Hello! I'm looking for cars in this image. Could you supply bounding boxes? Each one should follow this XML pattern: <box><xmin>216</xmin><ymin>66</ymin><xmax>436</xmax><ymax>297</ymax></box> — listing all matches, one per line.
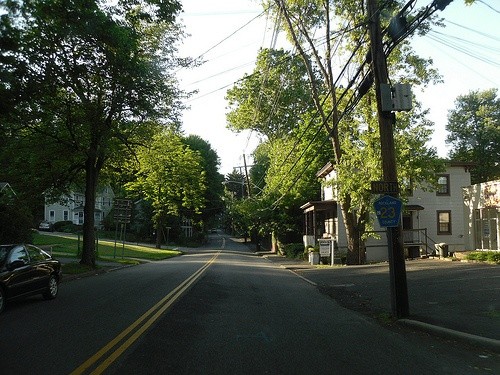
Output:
<box><xmin>0</xmin><ymin>244</ymin><xmax>61</xmax><ymax>310</ymax></box>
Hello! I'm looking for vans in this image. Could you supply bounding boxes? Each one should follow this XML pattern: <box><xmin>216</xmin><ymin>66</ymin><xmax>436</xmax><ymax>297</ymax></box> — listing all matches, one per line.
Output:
<box><xmin>212</xmin><ymin>228</ymin><xmax>217</xmax><ymax>233</ymax></box>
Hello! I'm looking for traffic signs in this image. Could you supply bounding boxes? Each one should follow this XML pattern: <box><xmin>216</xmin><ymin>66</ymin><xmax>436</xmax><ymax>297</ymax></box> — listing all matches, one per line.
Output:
<box><xmin>373</xmin><ymin>195</ymin><xmax>403</xmax><ymax>228</ymax></box>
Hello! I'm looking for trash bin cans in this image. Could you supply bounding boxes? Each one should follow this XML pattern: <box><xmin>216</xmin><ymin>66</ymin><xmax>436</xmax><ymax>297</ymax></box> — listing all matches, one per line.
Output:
<box><xmin>311</xmin><ymin>251</ymin><xmax>319</xmax><ymax>265</ymax></box>
<box><xmin>435</xmin><ymin>242</ymin><xmax>448</xmax><ymax>258</ymax></box>
